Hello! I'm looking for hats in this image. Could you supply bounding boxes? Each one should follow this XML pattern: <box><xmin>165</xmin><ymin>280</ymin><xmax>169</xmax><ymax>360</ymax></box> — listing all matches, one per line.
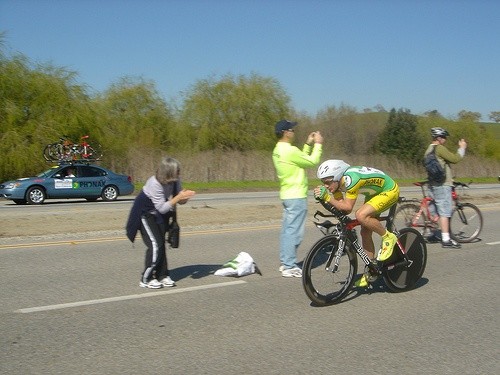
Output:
<box><xmin>275</xmin><ymin>119</ymin><xmax>298</xmax><ymax>134</ymax></box>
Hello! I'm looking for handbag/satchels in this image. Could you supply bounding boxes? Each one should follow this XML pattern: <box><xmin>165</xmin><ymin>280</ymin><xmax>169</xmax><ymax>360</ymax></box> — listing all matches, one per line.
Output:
<box><xmin>168</xmin><ymin>221</ymin><xmax>179</xmax><ymax>248</ymax></box>
<box><xmin>214</xmin><ymin>252</ymin><xmax>262</xmax><ymax>277</ymax></box>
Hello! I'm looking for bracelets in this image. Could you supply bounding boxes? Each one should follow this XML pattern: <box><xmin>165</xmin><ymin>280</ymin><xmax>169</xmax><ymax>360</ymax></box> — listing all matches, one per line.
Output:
<box><xmin>305</xmin><ymin>143</ymin><xmax>311</xmax><ymax>146</ymax></box>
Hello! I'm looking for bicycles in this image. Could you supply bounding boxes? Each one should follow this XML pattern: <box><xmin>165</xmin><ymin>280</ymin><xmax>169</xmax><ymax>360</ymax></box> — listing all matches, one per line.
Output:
<box><xmin>300</xmin><ymin>191</ymin><xmax>427</xmax><ymax>308</ymax></box>
<box><xmin>43</xmin><ymin>133</ymin><xmax>102</xmax><ymax>163</ymax></box>
<box><xmin>392</xmin><ymin>181</ymin><xmax>483</xmax><ymax>244</ymax></box>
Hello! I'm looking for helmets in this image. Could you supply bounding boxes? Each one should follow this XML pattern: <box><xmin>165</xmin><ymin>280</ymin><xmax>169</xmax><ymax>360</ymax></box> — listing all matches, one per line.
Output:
<box><xmin>317</xmin><ymin>160</ymin><xmax>351</xmax><ymax>182</ymax></box>
<box><xmin>431</xmin><ymin>127</ymin><xmax>449</xmax><ymax>138</ymax></box>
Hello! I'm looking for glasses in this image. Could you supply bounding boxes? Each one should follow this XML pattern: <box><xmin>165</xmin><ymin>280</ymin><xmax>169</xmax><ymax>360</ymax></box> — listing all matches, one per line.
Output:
<box><xmin>439</xmin><ymin>136</ymin><xmax>446</xmax><ymax>139</ymax></box>
<box><xmin>288</xmin><ymin>128</ymin><xmax>296</xmax><ymax>132</ymax></box>
<box><xmin>322</xmin><ymin>180</ymin><xmax>333</xmax><ymax>185</ymax></box>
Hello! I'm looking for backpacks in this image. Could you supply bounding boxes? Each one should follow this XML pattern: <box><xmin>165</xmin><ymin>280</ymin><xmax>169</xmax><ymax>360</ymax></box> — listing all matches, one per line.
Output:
<box><xmin>424</xmin><ymin>145</ymin><xmax>446</xmax><ymax>187</ymax></box>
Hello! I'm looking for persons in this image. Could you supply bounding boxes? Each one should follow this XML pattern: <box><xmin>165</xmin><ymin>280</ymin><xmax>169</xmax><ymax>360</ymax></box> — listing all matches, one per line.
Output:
<box><xmin>67</xmin><ymin>169</ymin><xmax>75</xmax><ymax>178</ymax></box>
<box><xmin>423</xmin><ymin>127</ymin><xmax>467</xmax><ymax>249</ymax></box>
<box><xmin>126</xmin><ymin>158</ymin><xmax>197</xmax><ymax>289</ymax></box>
<box><xmin>271</xmin><ymin>120</ymin><xmax>322</xmax><ymax>278</ymax></box>
<box><xmin>314</xmin><ymin>159</ymin><xmax>399</xmax><ymax>288</ymax></box>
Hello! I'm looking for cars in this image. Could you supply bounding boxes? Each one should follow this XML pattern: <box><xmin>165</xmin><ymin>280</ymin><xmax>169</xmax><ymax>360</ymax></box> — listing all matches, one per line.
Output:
<box><xmin>0</xmin><ymin>164</ymin><xmax>134</xmax><ymax>206</ymax></box>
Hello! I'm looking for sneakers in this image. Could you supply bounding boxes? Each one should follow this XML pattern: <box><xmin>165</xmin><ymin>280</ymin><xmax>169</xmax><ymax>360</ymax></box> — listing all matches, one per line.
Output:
<box><xmin>376</xmin><ymin>229</ymin><xmax>398</xmax><ymax>261</ymax></box>
<box><xmin>354</xmin><ymin>274</ymin><xmax>377</xmax><ymax>287</ymax></box>
<box><xmin>282</xmin><ymin>267</ymin><xmax>303</xmax><ymax>278</ymax></box>
<box><xmin>139</xmin><ymin>278</ymin><xmax>164</xmax><ymax>289</ymax></box>
<box><xmin>279</xmin><ymin>265</ymin><xmax>284</xmax><ymax>272</ymax></box>
<box><xmin>422</xmin><ymin>235</ymin><xmax>442</xmax><ymax>243</ymax></box>
<box><xmin>441</xmin><ymin>239</ymin><xmax>462</xmax><ymax>249</ymax></box>
<box><xmin>159</xmin><ymin>275</ymin><xmax>176</xmax><ymax>287</ymax></box>
